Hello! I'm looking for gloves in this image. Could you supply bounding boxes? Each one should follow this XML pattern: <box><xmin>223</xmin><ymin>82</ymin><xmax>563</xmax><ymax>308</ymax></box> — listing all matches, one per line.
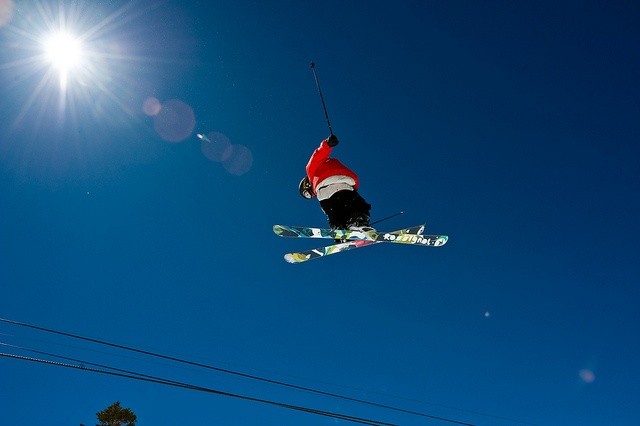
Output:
<box><xmin>327</xmin><ymin>134</ymin><xmax>339</xmax><ymax>147</ymax></box>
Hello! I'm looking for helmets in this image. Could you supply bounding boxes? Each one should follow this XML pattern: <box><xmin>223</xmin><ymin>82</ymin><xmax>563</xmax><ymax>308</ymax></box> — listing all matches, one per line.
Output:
<box><xmin>298</xmin><ymin>176</ymin><xmax>316</xmax><ymax>199</ymax></box>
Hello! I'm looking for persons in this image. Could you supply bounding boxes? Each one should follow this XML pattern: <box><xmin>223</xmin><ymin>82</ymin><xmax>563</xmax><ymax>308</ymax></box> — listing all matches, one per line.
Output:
<box><xmin>299</xmin><ymin>134</ymin><xmax>372</xmax><ymax>245</ymax></box>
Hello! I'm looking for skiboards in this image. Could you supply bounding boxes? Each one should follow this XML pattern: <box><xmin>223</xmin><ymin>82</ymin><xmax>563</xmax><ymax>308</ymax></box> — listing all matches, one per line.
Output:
<box><xmin>273</xmin><ymin>224</ymin><xmax>449</xmax><ymax>264</ymax></box>
